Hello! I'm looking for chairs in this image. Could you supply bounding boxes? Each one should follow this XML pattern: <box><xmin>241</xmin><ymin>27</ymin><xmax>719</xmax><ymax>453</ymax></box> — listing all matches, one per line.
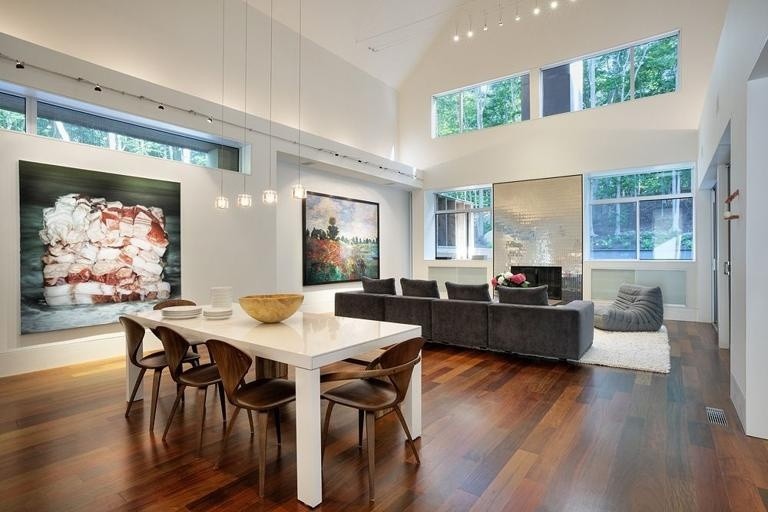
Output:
<box><xmin>152</xmin><ymin>326</ymin><xmax>255</xmax><ymax>459</ymax></box>
<box><xmin>151</xmin><ymin>299</ymin><xmax>217</xmax><ymax>401</ymax></box>
<box><xmin>118</xmin><ymin>317</ymin><xmax>200</xmax><ymax>430</ymax></box>
<box><xmin>204</xmin><ymin>337</ymin><xmax>296</xmax><ymax>497</ymax></box>
<box><xmin>320</xmin><ymin>336</ymin><xmax>427</xmax><ymax>502</ymax></box>
<box><xmin>593</xmin><ymin>282</ymin><xmax>664</xmax><ymax>331</ymax></box>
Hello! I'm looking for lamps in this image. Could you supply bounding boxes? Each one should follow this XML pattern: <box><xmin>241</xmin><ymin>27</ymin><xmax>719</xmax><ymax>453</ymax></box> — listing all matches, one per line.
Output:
<box><xmin>445</xmin><ymin>0</ymin><xmax>558</xmax><ymax>43</ymax></box>
<box><xmin>263</xmin><ymin>0</ymin><xmax>277</xmax><ymax>205</ymax></box>
<box><xmin>292</xmin><ymin>0</ymin><xmax>309</xmax><ymax>200</ymax></box>
<box><xmin>16</xmin><ymin>61</ymin><xmax>213</xmax><ymax>125</ymax></box>
<box><xmin>237</xmin><ymin>1</ymin><xmax>252</xmax><ymax>207</ymax></box>
<box><xmin>215</xmin><ymin>1</ymin><xmax>227</xmax><ymax>210</ymax></box>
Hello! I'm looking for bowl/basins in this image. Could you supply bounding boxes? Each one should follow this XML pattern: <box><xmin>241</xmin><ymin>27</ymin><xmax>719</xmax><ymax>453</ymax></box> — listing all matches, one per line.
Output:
<box><xmin>238</xmin><ymin>293</ymin><xmax>304</xmax><ymax>325</ymax></box>
<box><xmin>207</xmin><ymin>287</ymin><xmax>232</xmax><ymax>307</ymax></box>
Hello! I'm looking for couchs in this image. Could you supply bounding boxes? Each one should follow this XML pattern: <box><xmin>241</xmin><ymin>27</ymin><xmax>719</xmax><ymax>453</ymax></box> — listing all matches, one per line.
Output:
<box><xmin>335</xmin><ymin>290</ymin><xmax>595</xmax><ymax>361</ymax></box>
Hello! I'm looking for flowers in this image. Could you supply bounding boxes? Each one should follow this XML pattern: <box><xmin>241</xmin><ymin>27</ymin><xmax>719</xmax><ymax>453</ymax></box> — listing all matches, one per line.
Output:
<box><xmin>491</xmin><ymin>273</ymin><xmax>531</xmax><ymax>291</ymax></box>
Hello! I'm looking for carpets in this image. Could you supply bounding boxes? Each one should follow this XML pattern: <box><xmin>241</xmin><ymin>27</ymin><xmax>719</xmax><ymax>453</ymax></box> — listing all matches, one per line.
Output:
<box><xmin>421</xmin><ymin>290</ymin><xmax>672</xmax><ymax>374</ymax></box>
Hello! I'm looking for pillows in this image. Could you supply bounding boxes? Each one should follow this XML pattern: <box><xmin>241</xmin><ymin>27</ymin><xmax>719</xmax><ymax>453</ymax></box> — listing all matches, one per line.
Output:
<box><xmin>362</xmin><ymin>276</ymin><xmax>396</xmax><ymax>294</ymax></box>
<box><xmin>400</xmin><ymin>278</ymin><xmax>439</xmax><ymax>298</ymax></box>
<box><xmin>497</xmin><ymin>285</ymin><xmax>549</xmax><ymax>307</ymax></box>
<box><xmin>445</xmin><ymin>282</ymin><xmax>491</xmax><ymax>302</ymax></box>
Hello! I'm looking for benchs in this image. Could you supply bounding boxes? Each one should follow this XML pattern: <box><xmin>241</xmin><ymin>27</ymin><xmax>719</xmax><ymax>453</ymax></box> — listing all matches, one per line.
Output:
<box><xmin>253</xmin><ymin>347</ymin><xmax>401</xmax><ymax>421</ymax></box>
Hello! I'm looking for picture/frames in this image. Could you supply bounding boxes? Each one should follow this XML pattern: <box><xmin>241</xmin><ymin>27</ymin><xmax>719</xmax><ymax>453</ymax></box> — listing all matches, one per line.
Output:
<box><xmin>302</xmin><ymin>190</ymin><xmax>380</xmax><ymax>287</ymax></box>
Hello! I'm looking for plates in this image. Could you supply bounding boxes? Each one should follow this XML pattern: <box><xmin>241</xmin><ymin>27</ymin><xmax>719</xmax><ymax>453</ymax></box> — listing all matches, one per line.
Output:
<box><xmin>204</xmin><ymin>309</ymin><xmax>233</xmax><ymax>320</ymax></box>
<box><xmin>160</xmin><ymin>305</ymin><xmax>202</xmax><ymax>320</ymax></box>
